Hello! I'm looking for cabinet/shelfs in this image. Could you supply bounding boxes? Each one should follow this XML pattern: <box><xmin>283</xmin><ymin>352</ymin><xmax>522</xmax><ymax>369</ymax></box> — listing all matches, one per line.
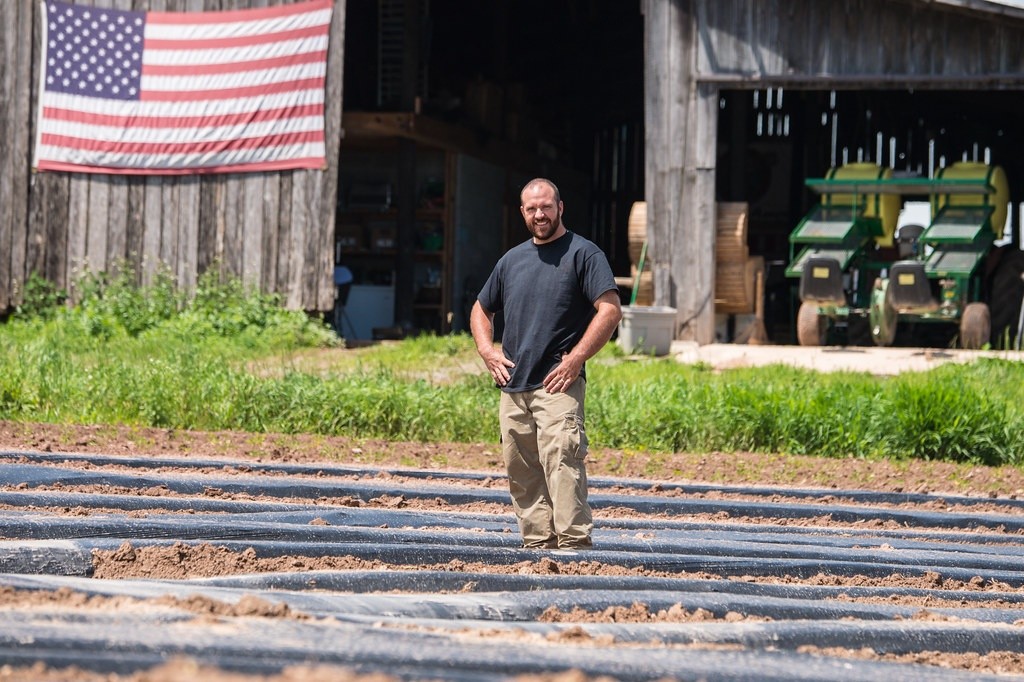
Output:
<box><xmin>341</xmin><ymin>154</ymin><xmax>445</xmax><ymax>336</ymax></box>
<box><xmin>335</xmin><ymin>269</ymin><xmax>397</xmax><ymax>341</ymax></box>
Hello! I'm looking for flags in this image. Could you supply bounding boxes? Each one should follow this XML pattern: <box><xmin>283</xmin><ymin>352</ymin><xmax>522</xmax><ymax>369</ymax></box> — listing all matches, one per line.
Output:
<box><xmin>31</xmin><ymin>0</ymin><xmax>335</xmax><ymax>174</ymax></box>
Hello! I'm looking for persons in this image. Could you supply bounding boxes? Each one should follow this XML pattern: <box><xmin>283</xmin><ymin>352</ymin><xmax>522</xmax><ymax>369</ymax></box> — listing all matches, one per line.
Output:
<box><xmin>469</xmin><ymin>178</ymin><xmax>623</xmax><ymax>552</ymax></box>
